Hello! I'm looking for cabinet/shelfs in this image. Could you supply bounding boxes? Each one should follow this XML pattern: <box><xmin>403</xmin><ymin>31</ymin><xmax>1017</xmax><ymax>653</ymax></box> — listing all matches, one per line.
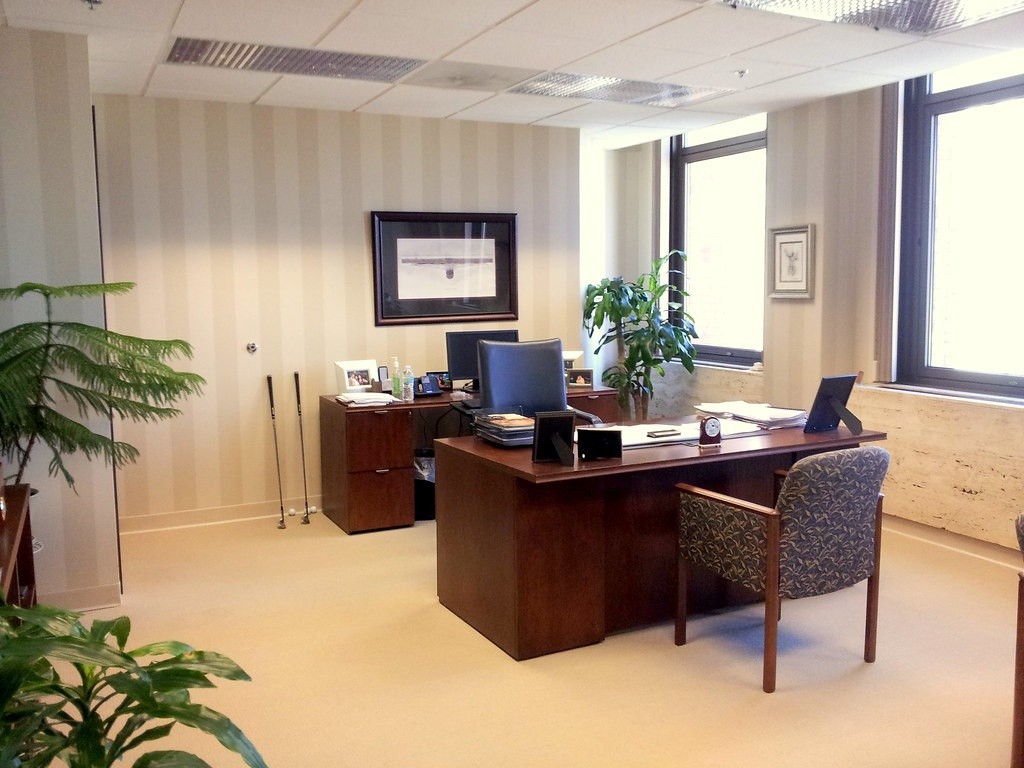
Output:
<box><xmin>0</xmin><ymin>483</ymin><xmax>37</xmax><ymax>628</ymax></box>
<box><xmin>319</xmin><ymin>388</ymin><xmax>618</xmax><ymax>536</ymax></box>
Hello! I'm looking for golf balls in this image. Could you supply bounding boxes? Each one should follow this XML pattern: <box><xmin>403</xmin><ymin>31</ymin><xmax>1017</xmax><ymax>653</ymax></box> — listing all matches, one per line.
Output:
<box><xmin>288</xmin><ymin>508</ymin><xmax>295</xmax><ymax>516</ymax></box>
<box><xmin>304</xmin><ymin>506</ymin><xmax>317</xmax><ymax>514</ymax></box>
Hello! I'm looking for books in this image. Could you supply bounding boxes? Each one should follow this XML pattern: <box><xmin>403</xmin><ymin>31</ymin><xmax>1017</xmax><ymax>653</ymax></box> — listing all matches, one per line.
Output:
<box><xmin>694</xmin><ymin>400</ymin><xmax>809</xmax><ymax>430</ymax></box>
<box><xmin>563</xmin><ymin>351</ymin><xmax>584</xmax><ymax>369</ymax></box>
<box><xmin>473</xmin><ymin>413</ymin><xmax>534</xmax><ymax>446</ymax></box>
<box><xmin>336</xmin><ymin>393</ymin><xmax>402</xmax><ymax>407</ymax></box>
<box><xmin>647</xmin><ymin>430</ymin><xmax>681</xmax><ymax>437</ymax></box>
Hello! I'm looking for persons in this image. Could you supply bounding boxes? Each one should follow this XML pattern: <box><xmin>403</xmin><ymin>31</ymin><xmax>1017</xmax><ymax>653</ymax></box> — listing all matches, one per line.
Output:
<box><xmin>576</xmin><ymin>374</ymin><xmax>585</xmax><ymax>384</ymax></box>
<box><xmin>439</xmin><ymin>375</ymin><xmax>447</xmax><ymax>387</ymax></box>
<box><xmin>349</xmin><ymin>373</ymin><xmax>368</xmax><ymax>385</ymax></box>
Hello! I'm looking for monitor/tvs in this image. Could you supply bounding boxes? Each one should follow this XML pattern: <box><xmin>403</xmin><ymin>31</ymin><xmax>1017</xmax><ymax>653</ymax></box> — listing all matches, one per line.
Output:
<box><xmin>446</xmin><ymin>330</ymin><xmax>519</xmax><ymax>394</ymax></box>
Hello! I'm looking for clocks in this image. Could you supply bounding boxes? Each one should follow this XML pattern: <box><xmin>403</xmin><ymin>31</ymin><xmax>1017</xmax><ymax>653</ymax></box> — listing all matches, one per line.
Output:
<box><xmin>698</xmin><ymin>416</ymin><xmax>721</xmax><ymax>449</ymax></box>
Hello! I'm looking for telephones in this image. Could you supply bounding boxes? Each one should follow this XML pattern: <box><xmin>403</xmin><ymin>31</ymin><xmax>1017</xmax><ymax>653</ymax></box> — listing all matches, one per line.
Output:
<box><xmin>413</xmin><ymin>375</ymin><xmax>444</xmax><ymax>397</ymax></box>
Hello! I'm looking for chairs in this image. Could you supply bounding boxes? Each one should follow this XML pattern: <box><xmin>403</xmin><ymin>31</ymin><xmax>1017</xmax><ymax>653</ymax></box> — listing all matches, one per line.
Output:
<box><xmin>478</xmin><ymin>338</ymin><xmax>602</xmax><ymax>424</ymax></box>
<box><xmin>674</xmin><ymin>445</ymin><xmax>891</xmax><ymax>694</ymax></box>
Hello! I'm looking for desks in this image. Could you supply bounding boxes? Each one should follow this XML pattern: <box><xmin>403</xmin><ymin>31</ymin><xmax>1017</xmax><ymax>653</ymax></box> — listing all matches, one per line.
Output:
<box><xmin>434</xmin><ymin>415</ymin><xmax>888</xmax><ymax>660</ymax></box>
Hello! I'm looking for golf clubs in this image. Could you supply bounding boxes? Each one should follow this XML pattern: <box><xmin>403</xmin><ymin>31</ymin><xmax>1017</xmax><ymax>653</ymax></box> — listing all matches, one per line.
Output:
<box><xmin>294</xmin><ymin>371</ymin><xmax>311</xmax><ymax>524</ymax></box>
<box><xmin>267</xmin><ymin>373</ymin><xmax>287</xmax><ymax>529</ymax></box>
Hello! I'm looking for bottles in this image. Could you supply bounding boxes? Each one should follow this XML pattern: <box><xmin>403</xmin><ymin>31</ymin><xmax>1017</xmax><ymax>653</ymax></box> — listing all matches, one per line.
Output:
<box><xmin>402</xmin><ymin>365</ymin><xmax>414</xmax><ymax>401</ymax></box>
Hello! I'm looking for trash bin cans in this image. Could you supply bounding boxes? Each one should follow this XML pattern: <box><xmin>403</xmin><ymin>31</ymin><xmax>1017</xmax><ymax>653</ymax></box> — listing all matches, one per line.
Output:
<box><xmin>414</xmin><ymin>447</ymin><xmax>436</xmax><ymax>521</ymax></box>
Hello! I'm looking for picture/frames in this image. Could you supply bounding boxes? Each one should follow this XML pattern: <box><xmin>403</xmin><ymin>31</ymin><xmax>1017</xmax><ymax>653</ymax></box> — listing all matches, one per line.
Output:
<box><xmin>767</xmin><ymin>223</ymin><xmax>815</xmax><ymax>299</ymax></box>
<box><xmin>370</xmin><ymin>210</ymin><xmax>519</xmax><ymax>326</ymax></box>
<box><xmin>334</xmin><ymin>359</ymin><xmax>378</xmax><ymax>395</ymax></box>
<box><xmin>566</xmin><ymin>368</ymin><xmax>593</xmax><ymax>389</ymax></box>
<box><xmin>427</xmin><ymin>371</ymin><xmax>452</xmax><ymax>391</ymax></box>
